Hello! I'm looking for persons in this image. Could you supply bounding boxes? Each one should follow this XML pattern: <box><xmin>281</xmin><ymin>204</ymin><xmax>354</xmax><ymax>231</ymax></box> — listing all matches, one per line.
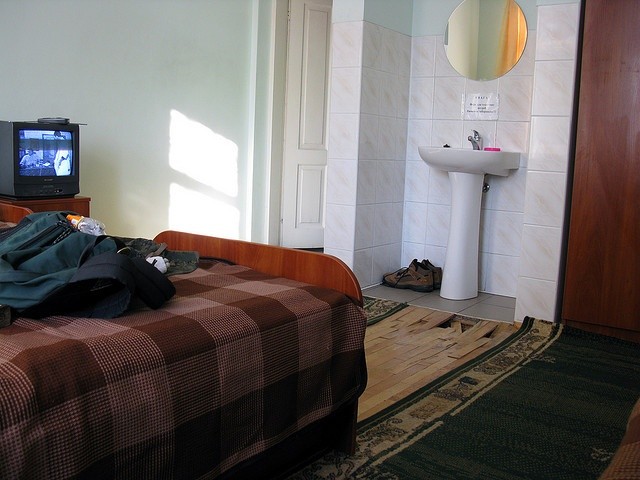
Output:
<box><xmin>20</xmin><ymin>147</ymin><xmax>40</xmax><ymax>168</ymax></box>
<box><xmin>53</xmin><ymin>130</ymin><xmax>71</xmax><ymax>176</ymax></box>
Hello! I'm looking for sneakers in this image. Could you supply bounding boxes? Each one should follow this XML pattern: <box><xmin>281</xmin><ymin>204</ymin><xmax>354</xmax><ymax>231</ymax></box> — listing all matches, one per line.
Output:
<box><xmin>421</xmin><ymin>259</ymin><xmax>442</xmax><ymax>291</ymax></box>
<box><xmin>383</xmin><ymin>258</ymin><xmax>434</xmax><ymax>293</ymax></box>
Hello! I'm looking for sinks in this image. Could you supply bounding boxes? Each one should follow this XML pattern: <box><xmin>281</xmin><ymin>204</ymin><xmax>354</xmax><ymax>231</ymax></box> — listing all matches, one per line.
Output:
<box><xmin>418</xmin><ymin>144</ymin><xmax>519</xmax><ymax>176</ymax></box>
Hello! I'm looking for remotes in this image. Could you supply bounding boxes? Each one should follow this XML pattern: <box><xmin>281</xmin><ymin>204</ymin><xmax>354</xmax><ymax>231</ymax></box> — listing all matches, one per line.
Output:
<box><xmin>37</xmin><ymin>117</ymin><xmax>71</xmax><ymax>122</ymax></box>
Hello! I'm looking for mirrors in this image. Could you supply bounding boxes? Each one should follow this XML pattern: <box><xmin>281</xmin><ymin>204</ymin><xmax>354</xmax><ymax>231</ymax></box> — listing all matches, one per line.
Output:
<box><xmin>443</xmin><ymin>0</ymin><xmax>528</xmax><ymax>81</ymax></box>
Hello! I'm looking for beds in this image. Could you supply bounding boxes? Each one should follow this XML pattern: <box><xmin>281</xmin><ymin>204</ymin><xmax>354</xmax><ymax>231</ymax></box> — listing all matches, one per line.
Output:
<box><xmin>2</xmin><ymin>200</ymin><xmax>362</xmax><ymax>478</ymax></box>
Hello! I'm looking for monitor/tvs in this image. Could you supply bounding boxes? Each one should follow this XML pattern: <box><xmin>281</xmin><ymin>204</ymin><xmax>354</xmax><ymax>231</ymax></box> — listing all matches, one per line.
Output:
<box><xmin>0</xmin><ymin>122</ymin><xmax>80</xmax><ymax>201</ymax></box>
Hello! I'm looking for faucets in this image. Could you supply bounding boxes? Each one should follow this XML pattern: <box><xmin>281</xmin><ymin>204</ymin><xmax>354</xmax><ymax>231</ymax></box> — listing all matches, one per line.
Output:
<box><xmin>468</xmin><ymin>128</ymin><xmax>482</xmax><ymax>150</ymax></box>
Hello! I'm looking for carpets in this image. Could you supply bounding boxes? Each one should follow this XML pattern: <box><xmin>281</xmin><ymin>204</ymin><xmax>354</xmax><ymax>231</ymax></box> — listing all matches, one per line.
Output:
<box><xmin>361</xmin><ymin>294</ymin><xmax>410</xmax><ymax>328</ymax></box>
<box><xmin>290</xmin><ymin>316</ymin><xmax>639</xmax><ymax>480</ymax></box>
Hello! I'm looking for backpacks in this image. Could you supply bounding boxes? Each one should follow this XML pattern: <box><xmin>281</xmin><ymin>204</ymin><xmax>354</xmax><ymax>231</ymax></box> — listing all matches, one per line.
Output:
<box><xmin>1</xmin><ymin>208</ymin><xmax>177</xmax><ymax>326</ymax></box>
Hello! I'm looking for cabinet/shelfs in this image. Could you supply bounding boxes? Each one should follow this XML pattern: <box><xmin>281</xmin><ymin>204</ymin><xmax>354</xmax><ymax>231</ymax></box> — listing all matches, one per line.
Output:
<box><xmin>2</xmin><ymin>195</ymin><xmax>91</xmax><ymax>216</ymax></box>
